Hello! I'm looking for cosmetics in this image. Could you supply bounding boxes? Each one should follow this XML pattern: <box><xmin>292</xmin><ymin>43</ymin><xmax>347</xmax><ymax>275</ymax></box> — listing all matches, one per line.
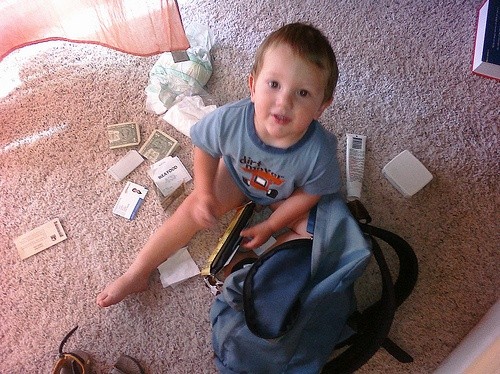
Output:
<box><xmin>381</xmin><ymin>149</ymin><xmax>434</xmax><ymax>199</ymax></box>
<box><xmin>344</xmin><ymin>131</ymin><xmax>370</xmax><ymax>202</ymax></box>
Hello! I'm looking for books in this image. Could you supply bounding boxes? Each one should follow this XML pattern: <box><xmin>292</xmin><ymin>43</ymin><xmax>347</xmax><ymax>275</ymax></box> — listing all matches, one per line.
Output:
<box><xmin>467</xmin><ymin>0</ymin><xmax>500</xmax><ymax>82</ymax></box>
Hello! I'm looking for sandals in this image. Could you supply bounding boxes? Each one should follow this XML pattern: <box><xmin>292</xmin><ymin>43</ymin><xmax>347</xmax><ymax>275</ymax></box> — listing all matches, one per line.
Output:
<box><xmin>44</xmin><ymin>326</ymin><xmax>91</xmax><ymax>374</ymax></box>
<box><xmin>107</xmin><ymin>355</ymin><xmax>142</xmax><ymax>374</ymax></box>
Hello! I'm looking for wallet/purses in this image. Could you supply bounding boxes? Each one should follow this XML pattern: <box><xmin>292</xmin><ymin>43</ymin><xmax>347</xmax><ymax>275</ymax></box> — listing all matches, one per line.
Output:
<box><xmin>202</xmin><ymin>199</ymin><xmax>257</xmax><ymax>283</ymax></box>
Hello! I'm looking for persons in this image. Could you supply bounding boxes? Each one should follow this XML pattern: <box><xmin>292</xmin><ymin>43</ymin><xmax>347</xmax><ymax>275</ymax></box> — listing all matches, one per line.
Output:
<box><xmin>94</xmin><ymin>19</ymin><xmax>344</xmax><ymax>308</ymax></box>
<box><xmin>131</xmin><ymin>188</ymin><xmax>145</xmax><ymax>197</ymax></box>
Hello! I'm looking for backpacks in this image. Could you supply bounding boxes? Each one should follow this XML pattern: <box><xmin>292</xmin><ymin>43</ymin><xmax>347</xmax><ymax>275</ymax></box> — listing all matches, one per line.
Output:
<box><xmin>200</xmin><ymin>200</ymin><xmax>420</xmax><ymax>374</ymax></box>
